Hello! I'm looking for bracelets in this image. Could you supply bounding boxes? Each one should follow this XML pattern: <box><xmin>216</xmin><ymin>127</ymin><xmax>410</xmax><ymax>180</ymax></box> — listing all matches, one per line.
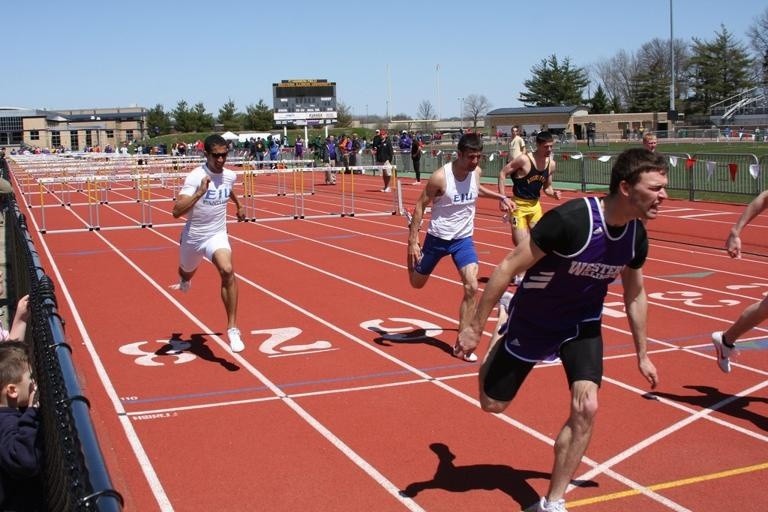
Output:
<box><xmin>499</xmin><ymin>194</ymin><xmax>507</xmax><ymax>202</ymax></box>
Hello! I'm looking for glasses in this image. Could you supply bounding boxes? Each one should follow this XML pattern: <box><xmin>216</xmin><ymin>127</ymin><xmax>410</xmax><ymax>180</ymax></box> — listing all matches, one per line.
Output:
<box><xmin>209</xmin><ymin>152</ymin><xmax>227</xmax><ymax>158</ymax></box>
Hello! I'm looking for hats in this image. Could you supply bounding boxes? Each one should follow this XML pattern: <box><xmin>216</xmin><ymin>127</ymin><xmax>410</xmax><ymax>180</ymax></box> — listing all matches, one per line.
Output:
<box><xmin>352</xmin><ymin>130</ymin><xmax>409</xmax><ymax>140</ymax></box>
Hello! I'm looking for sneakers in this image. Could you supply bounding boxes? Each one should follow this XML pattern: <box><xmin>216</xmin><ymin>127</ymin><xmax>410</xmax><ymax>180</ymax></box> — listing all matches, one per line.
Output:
<box><xmin>180</xmin><ymin>278</ymin><xmax>191</xmax><ymax>291</ymax></box>
<box><xmin>711</xmin><ymin>331</ymin><xmax>733</xmax><ymax>373</ymax></box>
<box><xmin>228</xmin><ymin>326</ymin><xmax>246</xmax><ymax>354</ymax></box>
<box><xmin>404</xmin><ymin>211</ymin><xmax>424</xmax><ymax>229</ymax></box>
<box><xmin>381</xmin><ymin>181</ymin><xmax>421</xmax><ymax>192</ymax></box>
<box><xmin>453</xmin><ymin>345</ymin><xmax>479</xmax><ymax>362</ymax></box>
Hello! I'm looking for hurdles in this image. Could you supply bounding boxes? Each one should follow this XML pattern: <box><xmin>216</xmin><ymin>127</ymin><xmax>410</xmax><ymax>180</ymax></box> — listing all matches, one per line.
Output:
<box><xmin>249</xmin><ymin>168</ymin><xmax>299</xmax><ymax>222</ymax></box>
<box><xmin>247</xmin><ymin>160</ymin><xmax>281</xmax><ymax>198</ymax></box>
<box><xmin>37</xmin><ymin>176</ymin><xmax>94</xmax><ymax>233</ymax></box>
<box><xmin>224</xmin><ymin>161</ymin><xmax>249</xmax><ymax>198</ymax></box>
<box><xmin>280</xmin><ymin>159</ymin><xmax>315</xmax><ymax>196</ymax></box>
<box><xmin>146</xmin><ymin>172</ymin><xmax>193</xmax><ymax>228</ymax></box>
<box><xmin>91</xmin><ymin>174</ymin><xmax>147</xmax><ymax>231</ymax></box>
<box><xmin>297</xmin><ymin>167</ymin><xmax>346</xmax><ymax>219</ymax></box>
<box><xmin>4</xmin><ymin>153</ymin><xmax>208</xmax><ymax>208</ymax></box>
<box><xmin>345</xmin><ymin>165</ymin><xmax>396</xmax><ymax>217</ymax></box>
<box><xmin>225</xmin><ymin>170</ymin><xmax>250</xmax><ymax>223</ymax></box>
<box><xmin>224</xmin><ymin>157</ymin><xmax>248</xmax><ymax>162</ymax></box>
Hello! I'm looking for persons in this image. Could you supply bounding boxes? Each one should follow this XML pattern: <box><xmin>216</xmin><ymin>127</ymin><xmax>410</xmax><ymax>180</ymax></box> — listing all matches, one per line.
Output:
<box><xmin>724</xmin><ymin>124</ymin><xmax>730</xmax><ymax>142</ymax></box>
<box><xmin>738</xmin><ymin>126</ymin><xmax>745</xmax><ymax>141</ymax></box>
<box><xmin>639</xmin><ymin>132</ymin><xmax>657</xmax><ymax>231</ymax></box>
<box><xmin>0</xmin><ymin>341</ymin><xmax>44</xmax><ymax>481</ymax></box>
<box><xmin>452</xmin><ymin>147</ymin><xmax>668</xmax><ymax>512</ymax></box>
<box><xmin>171</xmin><ymin>132</ymin><xmax>246</xmax><ymax>353</ymax></box>
<box><xmin>754</xmin><ymin>126</ymin><xmax>760</xmax><ymax>141</ymax></box>
<box><xmin>497</xmin><ymin>130</ymin><xmax>562</xmax><ymax>288</ymax></box>
<box><xmin>706</xmin><ymin>189</ymin><xmax>768</xmax><ymax>375</ymax></box>
<box><xmin>0</xmin><ymin>294</ymin><xmax>33</xmax><ymax>342</ymax></box>
<box><xmin>401</xmin><ymin>131</ymin><xmax>517</xmax><ymax>363</ymax></box>
<box><xmin>585</xmin><ymin>121</ymin><xmax>596</xmax><ymax>150</ymax></box>
